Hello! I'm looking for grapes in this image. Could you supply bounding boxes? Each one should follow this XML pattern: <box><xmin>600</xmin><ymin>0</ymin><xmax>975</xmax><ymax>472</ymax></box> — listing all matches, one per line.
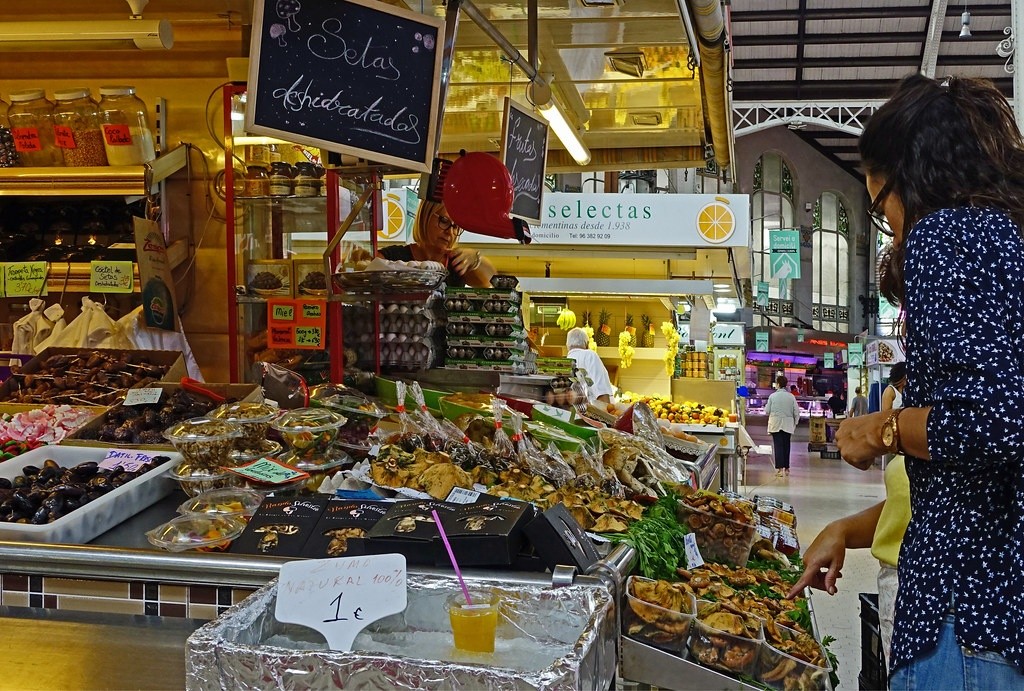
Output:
<box><xmin>618</xmin><ymin>330</ymin><xmax>634</xmax><ymax>368</ymax></box>
<box><xmin>661</xmin><ymin>321</ymin><xmax>679</xmax><ymax>377</ymax></box>
<box><xmin>584</xmin><ymin>327</ymin><xmax>597</xmax><ymax>351</ymax></box>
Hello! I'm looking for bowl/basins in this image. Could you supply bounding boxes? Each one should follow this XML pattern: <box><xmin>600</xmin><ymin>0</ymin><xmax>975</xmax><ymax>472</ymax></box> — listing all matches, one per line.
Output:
<box><xmin>144</xmin><ymin>383</ymin><xmax>387</xmax><ymax>553</ymax></box>
<box><xmin>621</xmin><ymin>573</ymin><xmax>833</xmax><ymax>691</ymax></box>
<box><xmin>375</xmin><ymin>374</ymin><xmax>600</xmax><ymax>455</ymax></box>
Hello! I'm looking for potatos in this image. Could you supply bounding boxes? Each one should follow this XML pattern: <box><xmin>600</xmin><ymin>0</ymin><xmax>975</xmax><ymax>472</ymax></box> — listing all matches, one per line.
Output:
<box><xmin>600</xmin><ymin>404</ymin><xmax>697</xmax><ymax>444</ymax></box>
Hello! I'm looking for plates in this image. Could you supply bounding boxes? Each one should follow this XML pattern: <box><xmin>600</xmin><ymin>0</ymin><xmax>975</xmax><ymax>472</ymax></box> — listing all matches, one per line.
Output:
<box><xmin>300</xmin><ymin>285</ymin><xmax>327</xmax><ymax>297</ymax></box>
<box><xmin>247</xmin><ymin>284</ymin><xmax>282</xmax><ymax>295</ymax></box>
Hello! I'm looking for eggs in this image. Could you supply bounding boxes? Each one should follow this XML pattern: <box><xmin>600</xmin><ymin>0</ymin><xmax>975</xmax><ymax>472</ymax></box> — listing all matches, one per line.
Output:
<box><xmin>343</xmin><ymin>302</ymin><xmax>427</xmax><ymax>359</ymax></box>
<box><xmin>545</xmin><ymin>390</ymin><xmax>576</xmax><ymax>406</ymax></box>
<box><xmin>382</xmin><ymin>259</ymin><xmax>446</xmax><ymax>271</ymax></box>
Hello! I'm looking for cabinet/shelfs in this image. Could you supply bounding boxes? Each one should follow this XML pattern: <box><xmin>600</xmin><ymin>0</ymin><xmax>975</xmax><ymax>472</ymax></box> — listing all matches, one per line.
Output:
<box><xmin>0</xmin><ymin>143</ymin><xmax>195</xmax><ymax>292</ymax></box>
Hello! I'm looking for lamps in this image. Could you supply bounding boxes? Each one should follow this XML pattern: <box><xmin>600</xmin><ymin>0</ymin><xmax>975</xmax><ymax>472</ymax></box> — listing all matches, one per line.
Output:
<box><xmin>0</xmin><ymin>0</ymin><xmax>176</xmax><ymax>52</ymax></box>
<box><xmin>529</xmin><ymin>84</ymin><xmax>592</xmax><ymax>166</ymax></box>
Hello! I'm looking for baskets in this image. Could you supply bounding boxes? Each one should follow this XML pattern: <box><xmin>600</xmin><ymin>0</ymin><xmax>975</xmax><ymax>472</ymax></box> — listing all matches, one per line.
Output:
<box><xmin>821</xmin><ymin>450</ymin><xmax>841</xmax><ymax>459</ymax></box>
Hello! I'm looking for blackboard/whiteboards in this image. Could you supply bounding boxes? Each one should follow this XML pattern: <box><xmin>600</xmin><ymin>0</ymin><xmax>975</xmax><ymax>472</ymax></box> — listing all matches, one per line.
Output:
<box><xmin>243</xmin><ymin>0</ymin><xmax>446</xmax><ymax>174</ymax></box>
<box><xmin>500</xmin><ymin>97</ymin><xmax>550</xmax><ymax>226</ymax></box>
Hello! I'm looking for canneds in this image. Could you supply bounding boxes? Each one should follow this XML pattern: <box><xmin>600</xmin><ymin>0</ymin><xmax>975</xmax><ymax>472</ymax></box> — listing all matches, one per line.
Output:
<box><xmin>717</xmin><ymin>354</ymin><xmax>738</xmax><ymax>382</ymax></box>
<box><xmin>0</xmin><ymin>86</ymin><xmax>156</xmax><ymax>168</ymax></box>
<box><xmin>677</xmin><ymin>350</ymin><xmax>708</xmax><ymax>378</ymax></box>
<box><xmin>244</xmin><ymin>161</ymin><xmax>364</xmax><ymax>198</ymax></box>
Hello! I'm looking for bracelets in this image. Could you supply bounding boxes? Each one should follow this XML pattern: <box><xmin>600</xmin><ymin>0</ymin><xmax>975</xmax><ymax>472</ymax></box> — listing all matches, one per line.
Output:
<box><xmin>472</xmin><ymin>251</ymin><xmax>482</xmax><ymax>270</ymax></box>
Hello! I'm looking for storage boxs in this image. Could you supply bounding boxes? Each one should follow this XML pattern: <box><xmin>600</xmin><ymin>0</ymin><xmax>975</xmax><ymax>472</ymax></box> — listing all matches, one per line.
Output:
<box><xmin>226</xmin><ymin>486</ymin><xmax>602</xmax><ymax>576</ymax></box>
<box><xmin>183</xmin><ymin>578</ymin><xmax>619</xmax><ymax>691</ymax></box>
<box><xmin>0</xmin><ymin>443</ymin><xmax>184</xmax><ymax>545</ymax></box>
<box><xmin>0</xmin><ymin>346</ymin><xmax>262</xmax><ymax>447</ymax></box>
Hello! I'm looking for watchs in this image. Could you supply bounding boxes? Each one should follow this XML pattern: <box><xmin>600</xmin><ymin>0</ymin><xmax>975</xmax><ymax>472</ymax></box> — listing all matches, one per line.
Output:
<box><xmin>881</xmin><ymin>407</ymin><xmax>905</xmax><ymax>456</ymax></box>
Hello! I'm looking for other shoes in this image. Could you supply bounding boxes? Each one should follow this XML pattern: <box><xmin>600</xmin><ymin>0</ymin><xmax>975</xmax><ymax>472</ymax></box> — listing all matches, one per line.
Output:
<box><xmin>784</xmin><ymin>467</ymin><xmax>789</xmax><ymax>476</ymax></box>
<box><xmin>775</xmin><ymin>471</ymin><xmax>783</xmax><ymax>477</ymax></box>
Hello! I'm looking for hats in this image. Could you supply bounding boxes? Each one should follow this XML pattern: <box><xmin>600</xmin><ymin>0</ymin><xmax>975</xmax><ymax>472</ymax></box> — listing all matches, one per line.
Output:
<box><xmin>890</xmin><ymin>362</ymin><xmax>907</xmax><ymax>387</ymax></box>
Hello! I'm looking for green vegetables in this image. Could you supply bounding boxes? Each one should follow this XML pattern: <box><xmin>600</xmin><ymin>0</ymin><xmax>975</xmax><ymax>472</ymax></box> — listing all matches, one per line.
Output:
<box><xmin>599</xmin><ymin>488</ymin><xmax>840</xmax><ymax>691</ymax></box>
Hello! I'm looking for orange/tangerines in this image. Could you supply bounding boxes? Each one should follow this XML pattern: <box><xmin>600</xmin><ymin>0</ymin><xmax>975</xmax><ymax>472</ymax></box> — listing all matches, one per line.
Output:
<box><xmin>621</xmin><ymin>390</ymin><xmax>728</xmax><ymax>425</ymax></box>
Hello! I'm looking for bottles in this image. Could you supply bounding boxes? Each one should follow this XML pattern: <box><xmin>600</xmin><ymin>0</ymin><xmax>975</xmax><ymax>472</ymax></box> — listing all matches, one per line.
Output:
<box><xmin>244</xmin><ymin>161</ymin><xmax>325</xmax><ymax>197</ymax></box>
<box><xmin>0</xmin><ymin>84</ymin><xmax>157</xmax><ymax>168</ymax></box>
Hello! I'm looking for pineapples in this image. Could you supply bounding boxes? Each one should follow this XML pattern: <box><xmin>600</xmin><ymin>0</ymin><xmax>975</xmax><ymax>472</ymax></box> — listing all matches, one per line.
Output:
<box><xmin>625</xmin><ymin>313</ymin><xmax>637</xmax><ymax>347</ymax></box>
<box><xmin>597</xmin><ymin>308</ymin><xmax>612</xmax><ymax>346</ymax></box>
<box><xmin>640</xmin><ymin>314</ymin><xmax>654</xmax><ymax>347</ymax></box>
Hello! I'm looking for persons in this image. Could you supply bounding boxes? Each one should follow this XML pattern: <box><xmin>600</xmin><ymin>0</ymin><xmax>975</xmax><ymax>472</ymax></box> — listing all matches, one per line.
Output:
<box><xmin>350</xmin><ymin>198</ymin><xmax>498</xmax><ymax>290</ymax></box>
<box><xmin>881</xmin><ymin>362</ymin><xmax>907</xmax><ymax>464</ymax></box>
<box><xmin>765</xmin><ymin>376</ymin><xmax>800</xmax><ymax>477</ymax></box>
<box><xmin>849</xmin><ymin>388</ymin><xmax>869</xmax><ymax>418</ymax></box>
<box><xmin>790</xmin><ymin>385</ymin><xmax>801</xmax><ymax>396</ymax></box>
<box><xmin>566</xmin><ymin>327</ymin><xmax>613</xmax><ymax>403</ymax></box>
<box><xmin>836</xmin><ymin>73</ymin><xmax>1024</xmax><ymax>691</ymax></box>
<box><xmin>786</xmin><ymin>244</ymin><xmax>912</xmax><ymax>677</ymax></box>
<box><xmin>827</xmin><ymin>389</ymin><xmax>847</xmax><ymax>419</ymax></box>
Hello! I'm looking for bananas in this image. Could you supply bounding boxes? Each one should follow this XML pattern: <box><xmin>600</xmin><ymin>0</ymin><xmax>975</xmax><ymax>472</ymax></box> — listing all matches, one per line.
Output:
<box><xmin>556</xmin><ymin>308</ymin><xmax>577</xmax><ymax>330</ymax></box>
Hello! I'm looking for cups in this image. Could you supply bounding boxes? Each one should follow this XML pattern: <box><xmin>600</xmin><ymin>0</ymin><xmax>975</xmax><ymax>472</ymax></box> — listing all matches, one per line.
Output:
<box><xmin>448</xmin><ymin>589</ymin><xmax>500</xmax><ymax>659</ymax></box>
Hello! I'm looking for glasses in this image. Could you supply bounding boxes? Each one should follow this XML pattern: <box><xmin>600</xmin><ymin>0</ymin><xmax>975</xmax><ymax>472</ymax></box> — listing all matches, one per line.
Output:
<box><xmin>433</xmin><ymin>213</ymin><xmax>465</xmax><ymax>236</ymax></box>
<box><xmin>865</xmin><ymin>176</ymin><xmax>899</xmax><ymax>236</ymax></box>
